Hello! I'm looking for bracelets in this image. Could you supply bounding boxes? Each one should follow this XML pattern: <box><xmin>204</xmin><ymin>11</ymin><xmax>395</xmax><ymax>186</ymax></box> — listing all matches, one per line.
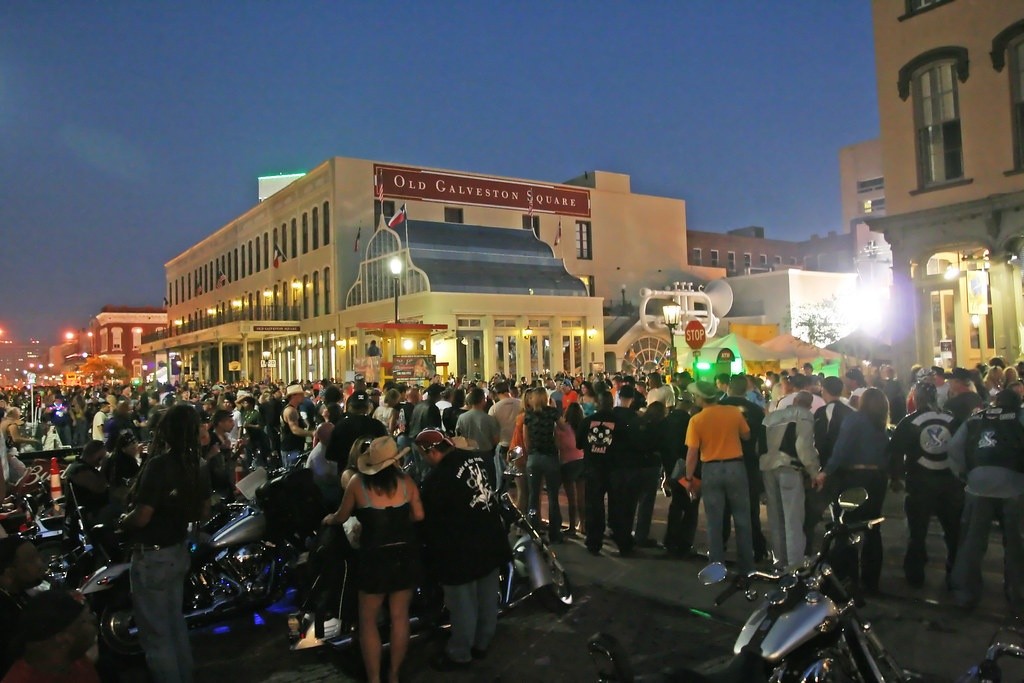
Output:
<box><xmin>118</xmin><ymin>516</ymin><xmax>124</xmax><ymax>527</ymax></box>
<box><xmin>686</xmin><ymin>477</ymin><xmax>693</xmax><ymax>482</ymax></box>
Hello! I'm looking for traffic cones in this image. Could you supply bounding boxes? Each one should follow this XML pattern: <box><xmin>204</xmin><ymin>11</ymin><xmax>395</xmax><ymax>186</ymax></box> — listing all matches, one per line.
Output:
<box><xmin>47</xmin><ymin>456</ymin><xmax>64</xmax><ymax>515</ymax></box>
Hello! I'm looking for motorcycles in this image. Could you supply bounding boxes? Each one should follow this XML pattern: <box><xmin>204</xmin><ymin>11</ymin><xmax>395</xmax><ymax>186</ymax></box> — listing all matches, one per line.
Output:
<box><xmin>0</xmin><ymin>446</ymin><xmax>582</xmax><ymax>683</ymax></box>
<box><xmin>584</xmin><ymin>486</ymin><xmax>1024</xmax><ymax>683</ymax></box>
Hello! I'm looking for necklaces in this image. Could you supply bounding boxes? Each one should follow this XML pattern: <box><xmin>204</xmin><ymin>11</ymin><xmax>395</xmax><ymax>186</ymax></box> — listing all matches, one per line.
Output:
<box><xmin>0</xmin><ymin>588</ymin><xmax>23</xmax><ymax>610</ymax></box>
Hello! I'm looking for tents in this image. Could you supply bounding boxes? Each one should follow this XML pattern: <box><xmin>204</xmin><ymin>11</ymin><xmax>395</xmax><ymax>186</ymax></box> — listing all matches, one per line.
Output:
<box><xmin>758</xmin><ymin>332</ymin><xmax>866</xmax><ymax>377</ymax></box>
<box><xmin>679</xmin><ymin>332</ymin><xmax>799</xmax><ymax>381</ymax></box>
<box><xmin>824</xmin><ymin>328</ymin><xmax>894</xmax><ymax>367</ymax></box>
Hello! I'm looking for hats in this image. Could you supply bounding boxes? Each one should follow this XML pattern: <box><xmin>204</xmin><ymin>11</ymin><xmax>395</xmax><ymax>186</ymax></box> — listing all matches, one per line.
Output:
<box><xmin>414</xmin><ymin>430</ymin><xmax>445</xmax><ymax>447</ymax></box>
<box><xmin>688</xmin><ymin>381</ymin><xmax>716</xmax><ymax>399</ymax></box>
<box><xmin>285</xmin><ymin>385</ymin><xmax>306</xmax><ymax>398</ymax></box>
<box><xmin>943</xmin><ymin>366</ymin><xmax>969</xmax><ymax>380</ymax></box>
<box><xmin>357</xmin><ymin>436</ymin><xmax>411</xmax><ymax>475</ymax></box>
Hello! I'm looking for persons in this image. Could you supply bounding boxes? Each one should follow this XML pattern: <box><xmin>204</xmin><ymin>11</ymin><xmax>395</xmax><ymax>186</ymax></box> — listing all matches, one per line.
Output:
<box><xmin>0</xmin><ymin>362</ymin><xmax>1024</xmax><ymax>683</ymax></box>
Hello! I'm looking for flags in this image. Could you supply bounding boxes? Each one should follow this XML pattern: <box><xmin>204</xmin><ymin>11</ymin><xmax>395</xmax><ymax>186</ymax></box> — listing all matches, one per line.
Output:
<box><xmin>163</xmin><ymin>297</ymin><xmax>168</xmax><ymax>305</ymax></box>
<box><xmin>217</xmin><ymin>271</ymin><xmax>226</xmax><ymax>289</ymax></box>
<box><xmin>355</xmin><ymin>232</ymin><xmax>360</xmax><ymax>251</ymax></box>
<box><xmin>273</xmin><ymin>244</ymin><xmax>282</xmax><ymax>268</ymax></box>
<box><xmin>197</xmin><ymin>283</ymin><xmax>202</xmax><ymax>291</ymax></box>
<box><xmin>379</xmin><ymin>183</ymin><xmax>384</xmax><ymax>201</ymax></box>
<box><xmin>388</xmin><ymin>203</ymin><xmax>407</xmax><ymax>227</ymax></box>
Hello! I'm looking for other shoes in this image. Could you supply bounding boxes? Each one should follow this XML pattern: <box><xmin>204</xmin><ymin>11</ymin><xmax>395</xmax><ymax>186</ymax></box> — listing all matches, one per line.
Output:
<box><xmin>539</xmin><ymin>520</ymin><xmax>767</xmax><ymax>563</ymax></box>
<box><xmin>430</xmin><ymin>650</ymin><xmax>471</xmax><ymax>670</ymax></box>
<box><xmin>469</xmin><ymin>647</ymin><xmax>488</xmax><ymax>659</ymax></box>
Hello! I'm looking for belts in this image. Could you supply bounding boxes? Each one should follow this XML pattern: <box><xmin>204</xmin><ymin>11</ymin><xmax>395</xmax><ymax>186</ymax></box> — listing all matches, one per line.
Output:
<box><xmin>707</xmin><ymin>457</ymin><xmax>743</xmax><ymax>464</ymax></box>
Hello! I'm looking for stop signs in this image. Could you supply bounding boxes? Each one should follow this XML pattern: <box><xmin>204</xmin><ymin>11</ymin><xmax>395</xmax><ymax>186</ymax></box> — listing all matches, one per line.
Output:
<box><xmin>685</xmin><ymin>320</ymin><xmax>706</xmax><ymax>349</ymax></box>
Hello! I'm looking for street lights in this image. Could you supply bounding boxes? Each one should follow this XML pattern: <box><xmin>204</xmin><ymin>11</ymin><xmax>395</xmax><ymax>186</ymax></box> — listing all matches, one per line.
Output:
<box><xmin>389</xmin><ymin>256</ymin><xmax>405</xmax><ymax>324</ymax></box>
<box><xmin>661</xmin><ymin>298</ymin><xmax>682</xmax><ymax>382</ymax></box>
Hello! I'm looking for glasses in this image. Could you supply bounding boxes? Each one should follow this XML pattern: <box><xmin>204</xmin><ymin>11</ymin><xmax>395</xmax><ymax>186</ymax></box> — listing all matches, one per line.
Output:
<box><xmin>361</xmin><ymin>440</ymin><xmax>371</xmax><ymax>452</ymax></box>
